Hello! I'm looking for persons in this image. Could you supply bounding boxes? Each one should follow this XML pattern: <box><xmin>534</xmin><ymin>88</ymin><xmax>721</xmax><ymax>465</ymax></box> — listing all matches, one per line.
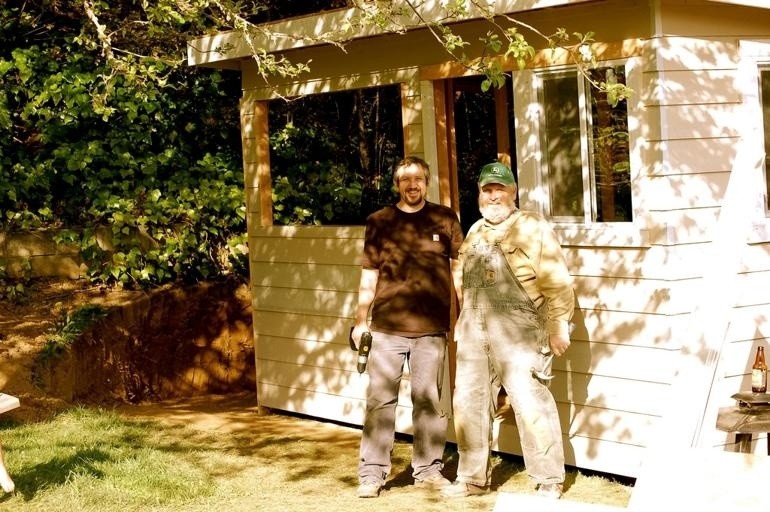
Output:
<box><xmin>438</xmin><ymin>163</ymin><xmax>578</xmax><ymax>504</ymax></box>
<box><xmin>346</xmin><ymin>153</ymin><xmax>466</xmax><ymax>502</ymax></box>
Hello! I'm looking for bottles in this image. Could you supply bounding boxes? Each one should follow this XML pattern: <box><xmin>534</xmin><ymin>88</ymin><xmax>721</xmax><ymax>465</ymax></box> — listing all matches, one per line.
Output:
<box><xmin>751</xmin><ymin>345</ymin><xmax>768</xmax><ymax>393</ymax></box>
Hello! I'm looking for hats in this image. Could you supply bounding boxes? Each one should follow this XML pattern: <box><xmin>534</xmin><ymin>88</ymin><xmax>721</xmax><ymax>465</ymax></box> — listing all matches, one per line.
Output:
<box><xmin>479</xmin><ymin>163</ymin><xmax>516</xmax><ymax>188</ymax></box>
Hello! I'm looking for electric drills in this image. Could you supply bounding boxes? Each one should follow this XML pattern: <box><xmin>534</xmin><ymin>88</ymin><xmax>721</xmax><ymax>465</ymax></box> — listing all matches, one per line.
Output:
<box><xmin>349</xmin><ymin>326</ymin><xmax>373</xmax><ymax>378</ymax></box>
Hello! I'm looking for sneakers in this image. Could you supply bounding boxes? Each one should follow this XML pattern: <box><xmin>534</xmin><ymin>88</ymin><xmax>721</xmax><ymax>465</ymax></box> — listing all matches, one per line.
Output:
<box><xmin>357</xmin><ymin>480</ymin><xmax>381</xmax><ymax>498</ymax></box>
<box><xmin>415</xmin><ymin>476</ymin><xmax>451</xmax><ymax>486</ymax></box>
<box><xmin>537</xmin><ymin>482</ymin><xmax>564</xmax><ymax>500</ymax></box>
<box><xmin>441</xmin><ymin>482</ymin><xmax>491</xmax><ymax>496</ymax></box>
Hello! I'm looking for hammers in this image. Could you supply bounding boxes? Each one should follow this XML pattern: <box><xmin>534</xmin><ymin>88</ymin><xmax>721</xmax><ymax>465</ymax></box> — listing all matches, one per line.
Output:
<box><xmin>529</xmin><ymin>323</ymin><xmax>575</xmax><ymax>381</ymax></box>
